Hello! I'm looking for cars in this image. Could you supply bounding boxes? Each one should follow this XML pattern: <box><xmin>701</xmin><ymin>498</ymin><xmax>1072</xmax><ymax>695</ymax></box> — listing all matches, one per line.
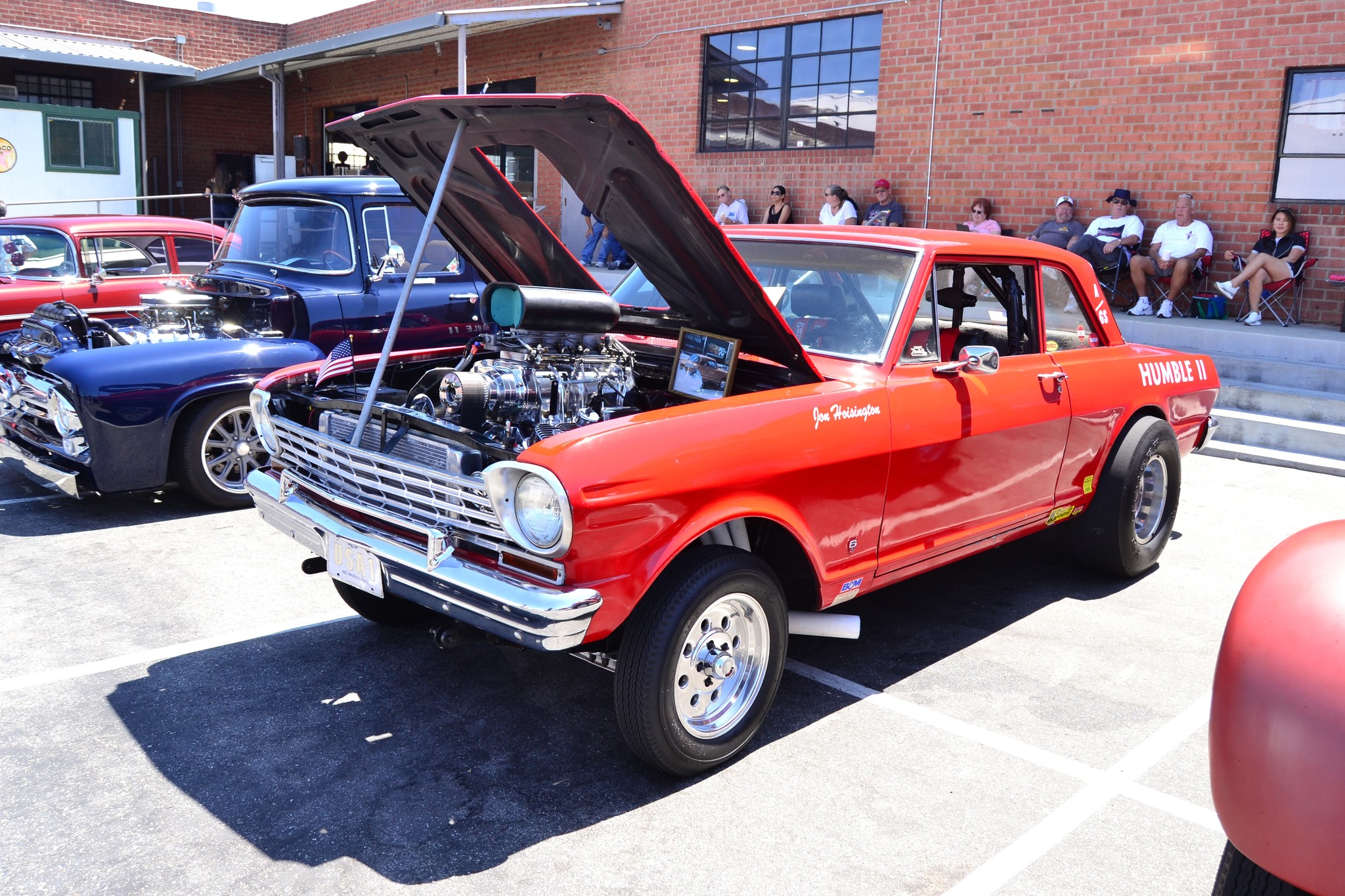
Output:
<box><xmin>0</xmin><ymin>166</ymin><xmax>489</xmax><ymax>512</ymax></box>
<box><xmin>0</xmin><ymin>212</ymin><xmax>240</xmax><ymax>340</ymax></box>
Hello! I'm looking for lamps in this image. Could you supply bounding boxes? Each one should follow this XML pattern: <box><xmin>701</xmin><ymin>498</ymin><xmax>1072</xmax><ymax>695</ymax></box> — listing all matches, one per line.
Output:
<box><xmin>297</xmin><ymin>69</ymin><xmax>303</xmax><ymax>82</ymax></box>
<box><xmin>434</xmin><ymin>41</ymin><xmax>442</xmax><ymax>56</ymax></box>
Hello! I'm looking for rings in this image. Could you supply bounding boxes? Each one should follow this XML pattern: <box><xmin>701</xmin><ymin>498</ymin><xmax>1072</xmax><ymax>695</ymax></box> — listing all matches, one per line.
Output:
<box><xmin>1108</xmin><ymin>248</ymin><xmax>1111</xmax><ymax>250</ymax></box>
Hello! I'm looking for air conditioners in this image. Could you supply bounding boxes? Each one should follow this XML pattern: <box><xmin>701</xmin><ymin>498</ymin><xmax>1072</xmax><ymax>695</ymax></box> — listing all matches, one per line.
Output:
<box><xmin>0</xmin><ymin>84</ymin><xmax>20</xmax><ymax>102</ymax></box>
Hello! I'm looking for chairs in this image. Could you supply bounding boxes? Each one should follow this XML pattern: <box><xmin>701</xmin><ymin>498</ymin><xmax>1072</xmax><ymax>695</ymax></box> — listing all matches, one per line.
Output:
<box><xmin>1000</xmin><ymin>228</ymin><xmax>1015</xmax><ymax>238</ymax></box>
<box><xmin>1135</xmin><ymin>227</ymin><xmax>1213</xmax><ymax>317</ymax></box>
<box><xmin>1231</xmin><ymin>228</ymin><xmax>1319</xmax><ymax>328</ymax></box>
<box><xmin>416</xmin><ymin>240</ymin><xmax>457</xmax><ymax>274</ymax></box>
<box><xmin>788</xmin><ymin>281</ymin><xmax>858</xmax><ymax>356</ymax></box>
<box><xmin>1325</xmin><ymin>274</ymin><xmax>1345</xmax><ymax>333</ymax></box>
<box><xmin>1056</xmin><ymin>241</ymin><xmax>1142</xmax><ymax>310</ymax></box>
<box><xmin>369</xmin><ymin>237</ymin><xmax>411</xmax><ymax>275</ymax></box>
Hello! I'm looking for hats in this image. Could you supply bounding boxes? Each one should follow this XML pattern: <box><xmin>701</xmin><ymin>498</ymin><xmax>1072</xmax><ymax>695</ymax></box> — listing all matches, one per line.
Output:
<box><xmin>1105</xmin><ymin>188</ymin><xmax>1137</xmax><ymax>207</ymax></box>
<box><xmin>1056</xmin><ymin>196</ymin><xmax>1073</xmax><ymax>208</ymax></box>
<box><xmin>875</xmin><ymin>179</ymin><xmax>890</xmax><ymax>189</ymax></box>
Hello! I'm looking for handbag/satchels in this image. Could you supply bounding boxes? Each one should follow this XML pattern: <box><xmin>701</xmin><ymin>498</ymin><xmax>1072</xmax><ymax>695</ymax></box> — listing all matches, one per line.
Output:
<box><xmin>978</xmin><ymin>274</ymin><xmax>1003</xmax><ymax>302</ymax></box>
<box><xmin>1190</xmin><ymin>296</ymin><xmax>1229</xmax><ymax>320</ymax></box>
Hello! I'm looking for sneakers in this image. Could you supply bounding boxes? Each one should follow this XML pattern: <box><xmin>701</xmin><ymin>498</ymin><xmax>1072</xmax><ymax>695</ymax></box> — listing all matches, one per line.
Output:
<box><xmin>1244</xmin><ymin>311</ymin><xmax>1262</xmax><ymax>326</ymax></box>
<box><xmin>1126</xmin><ymin>298</ymin><xmax>1153</xmax><ymax>316</ymax></box>
<box><xmin>1156</xmin><ymin>300</ymin><xmax>1173</xmax><ymax>318</ymax></box>
<box><xmin>1214</xmin><ymin>281</ymin><xmax>1240</xmax><ymax>299</ymax></box>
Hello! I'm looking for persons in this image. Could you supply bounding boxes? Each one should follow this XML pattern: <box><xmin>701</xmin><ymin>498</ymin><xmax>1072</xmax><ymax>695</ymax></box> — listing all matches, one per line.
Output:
<box><xmin>204</xmin><ymin>163</ymin><xmax>254</xmax><ymax>228</ymax></box>
<box><xmin>1024</xmin><ymin>196</ymin><xmax>1086</xmax><ymax>251</ymax></box>
<box><xmin>1214</xmin><ymin>208</ymin><xmax>1306</xmax><ymax>326</ymax></box>
<box><xmin>360</xmin><ymin>160</ymin><xmax>387</xmax><ymax>175</ymax></box>
<box><xmin>948</xmin><ymin>196</ymin><xmax>1000</xmax><ymax>291</ymax></box>
<box><xmin>818</xmin><ymin>185</ymin><xmax>859</xmax><ymax>225</ymax></box>
<box><xmin>714</xmin><ymin>185</ymin><xmax>749</xmax><ymax>225</ymax></box>
<box><xmin>579</xmin><ymin>203</ymin><xmax>632</xmax><ymax>271</ymax></box>
<box><xmin>761</xmin><ymin>185</ymin><xmax>793</xmax><ymax>224</ymax></box>
<box><xmin>1127</xmin><ymin>193</ymin><xmax>1213</xmax><ymax>319</ymax></box>
<box><xmin>1040</xmin><ymin>188</ymin><xmax>1144</xmax><ymax>314</ymax></box>
<box><xmin>861</xmin><ymin>179</ymin><xmax>904</xmax><ymax>227</ymax></box>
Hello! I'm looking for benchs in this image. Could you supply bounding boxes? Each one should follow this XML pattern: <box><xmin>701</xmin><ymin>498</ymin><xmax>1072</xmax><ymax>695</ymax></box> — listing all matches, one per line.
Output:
<box><xmin>854</xmin><ymin>314</ymin><xmax>1091</xmax><ymax>363</ymax></box>
<box><xmin>143</xmin><ymin>261</ymin><xmax>210</xmax><ymax>276</ymax></box>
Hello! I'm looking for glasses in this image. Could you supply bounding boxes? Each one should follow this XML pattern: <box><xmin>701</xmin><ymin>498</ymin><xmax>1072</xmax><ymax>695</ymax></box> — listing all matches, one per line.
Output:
<box><xmin>1058</xmin><ymin>206</ymin><xmax>1073</xmax><ymax>211</ymax></box>
<box><xmin>972</xmin><ymin>209</ymin><xmax>985</xmax><ymax>214</ymax></box>
<box><xmin>1112</xmin><ymin>199</ymin><xmax>1129</xmax><ymax>205</ymax></box>
<box><xmin>717</xmin><ymin>191</ymin><xmax>728</xmax><ymax>199</ymax></box>
<box><xmin>875</xmin><ymin>188</ymin><xmax>888</xmax><ymax>194</ymax></box>
<box><xmin>825</xmin><ymin>193</ymin><xmax>834</xmax><ymax>196</ymax></box>
<box><xmin>771</xmin><ymin>191</ymin><xmax>781</xmax><ymax>196</ymax></box>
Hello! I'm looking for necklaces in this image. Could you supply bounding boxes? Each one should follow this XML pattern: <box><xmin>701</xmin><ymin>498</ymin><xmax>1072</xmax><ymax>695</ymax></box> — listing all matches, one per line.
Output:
<box><xmin>832</xmin><ymin>203</ymin><xmax>840</xmax><ymax>209</ymax></box>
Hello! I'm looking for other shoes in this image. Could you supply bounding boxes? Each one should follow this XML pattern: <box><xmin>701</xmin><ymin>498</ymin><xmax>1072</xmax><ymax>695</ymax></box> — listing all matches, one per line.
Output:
<box><xmin>595</xmin><ymin>261</ymin><xmax>607</xmax><ymax>267</ymax></box>
<box><xmin>1063</xmin><ymin>298</ymin><xmax>1078</xmax><ymax>313</ymax></box>
<box><xmin>606</xmin><ymin>261</ymin><xmax>618</xmax><ymax>270</ymax></box>
<box><xmin>754</xmin><ymin>271</ymin><xmax>764</xmax><ymax>281</ymax></box>
<box><xmin>579</xmin><ymin>260</ymin><xmax>591</xmax><ymax>266</ymax></box>
<box><xmin>1021</xmin><ymin>291</ymin><xmax>1026</xmax><ymax>304</ymax></box>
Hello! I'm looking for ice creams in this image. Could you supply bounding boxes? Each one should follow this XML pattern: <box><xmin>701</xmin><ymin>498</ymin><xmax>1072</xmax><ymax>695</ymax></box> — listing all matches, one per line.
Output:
<box><xmin>721</xmin><ymin>212</ymin><xmax>725</xmax><ymax>217</ymax></box>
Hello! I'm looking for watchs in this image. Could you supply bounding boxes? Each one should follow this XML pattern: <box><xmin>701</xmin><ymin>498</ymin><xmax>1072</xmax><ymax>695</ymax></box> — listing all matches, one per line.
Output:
<box><xmin>732</xmin><ymin>220</ymin><xmax>736</xmax><ymax>224</ymax></box>
<box><xmin>1117</xmin><ymin>238</ymin><xmax>1121</xmax><ymax>245</ymax></box>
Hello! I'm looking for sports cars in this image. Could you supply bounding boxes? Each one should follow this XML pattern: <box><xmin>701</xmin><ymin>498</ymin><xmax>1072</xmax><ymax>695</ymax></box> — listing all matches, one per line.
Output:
<box><xmin>238</xmin><ymin>91</ymin><xmax>1224</xmax><ymax>773</ymax></box>
